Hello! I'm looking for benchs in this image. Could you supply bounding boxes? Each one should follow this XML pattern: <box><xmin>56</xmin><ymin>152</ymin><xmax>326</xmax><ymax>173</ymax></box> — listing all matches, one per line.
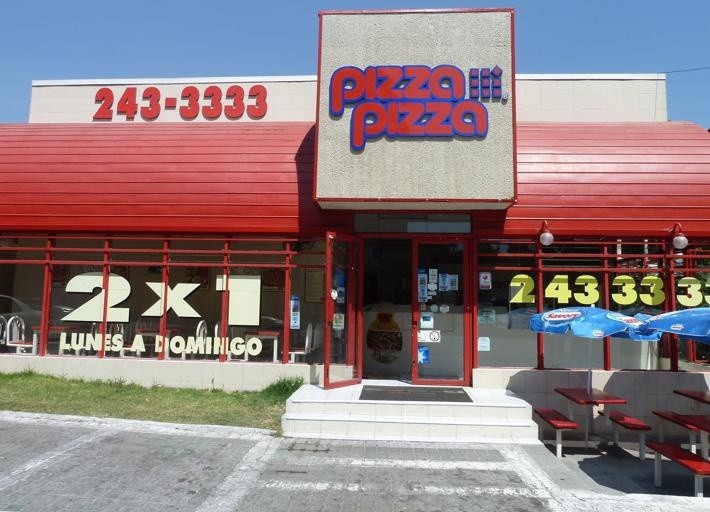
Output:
<box><xmin>644</xmin><ymin>440</ymin><xmax>710</xmax><ymax>497</ymax></box>
<box><xmin>598</xmin><ymin>409</ymin><xmax>652</xmax><ymax>460</ymax></box>
<box><xmin>653</xmin><ymin>408</ymin><xmax>702</xmax><ymax>455</ymax></box>
<box><xmin>534</xmin><ymin>406</ymin><xmax>581</xmax><ymax>458</ymax></box>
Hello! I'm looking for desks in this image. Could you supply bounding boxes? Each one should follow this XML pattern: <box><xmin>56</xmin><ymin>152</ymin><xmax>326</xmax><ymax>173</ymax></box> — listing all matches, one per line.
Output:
<box><xmin>31</xmin><ymin>325</ymin><xmax>89</xmax><ymax>356</ymax></box>
<box><xmin>673</xmin><ymin>413</ymin><xmax>710</xmax><ymax>458</ymax></box>
<box><xmin>673</xmin><ymin>389</ymin><xmax>710</xmax><ymax>458</ymax></box>
<box><xmin>135</xmin><ymin>326</ymin><xmax>194</xmax><ymax>359</ymax></box>
<box><xmin>244</xmin><ymin>331</ymin><xmax>281</xmax><ymax>363</ymax></box>
<box><xmin>553</xmin><ymin>387</ymin><xmax>627</xmax><ymax>455</ymax></box>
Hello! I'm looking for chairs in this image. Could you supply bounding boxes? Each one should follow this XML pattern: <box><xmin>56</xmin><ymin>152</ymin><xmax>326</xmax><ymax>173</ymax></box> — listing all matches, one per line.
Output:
<box><xmin>280</xmin><ymin>324</ymin><xmax>319</xmax><ymax>364</ymax></box>
<box><xmin>182</xmin><ymin>320</ymin><xmax>207</xmax><ymax>361</ymax></box>
<box><xmin>109</xmin><ymin>324</ymin><xmax>136</xmax><ymax>357</ymax></box>
<box><xmin>6</xmin><ymin>316</ymin><xmax>33</xmax><ymax>354</ymax></box>
<box><xmin>214</xmin><ymin>321</ymin><xmax>241</xmax><ymax>361</ymax></box>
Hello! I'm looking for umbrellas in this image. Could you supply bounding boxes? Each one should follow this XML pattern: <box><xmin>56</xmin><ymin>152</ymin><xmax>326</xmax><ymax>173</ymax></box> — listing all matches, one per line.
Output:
<box><xmin>633</xmin><ymin>307</ymin><xmax>710</xmax><ymax>347</ymax></box>
<box><xmin>527</xmin><ymin>303</ymin><xmax>663</xmax><ymax>394</ymax></box>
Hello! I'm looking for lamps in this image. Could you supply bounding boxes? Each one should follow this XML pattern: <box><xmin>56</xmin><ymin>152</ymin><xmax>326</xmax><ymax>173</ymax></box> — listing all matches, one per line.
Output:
<box><xmin>539</xmin><ymin>220</ymin><xmax>554</xmax><ymax>246</ymax></box>
<box><xmin>671</xmin><ymin>223</ymin><xmax>688</xmax><ymax>249</ymax></box>
